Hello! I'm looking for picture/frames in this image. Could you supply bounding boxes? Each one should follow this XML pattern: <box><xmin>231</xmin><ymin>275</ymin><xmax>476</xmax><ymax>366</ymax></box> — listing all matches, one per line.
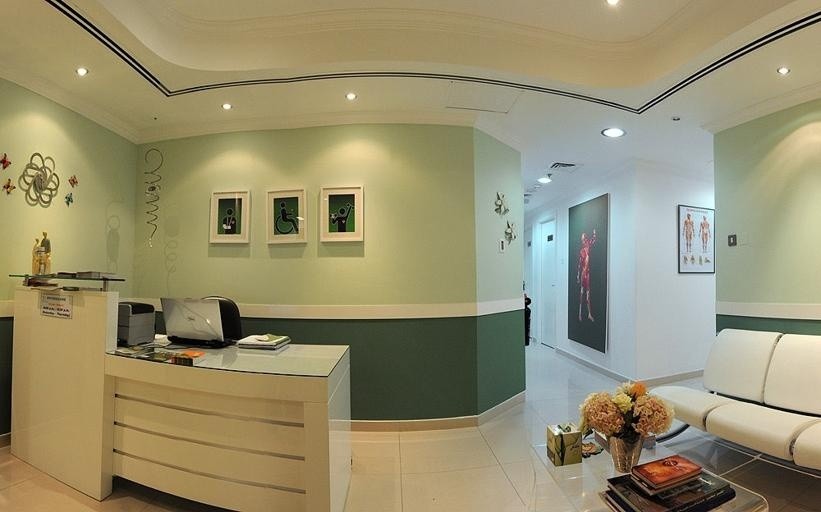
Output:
<box><xmin>209</xmin><ymin>186</ymin><xmax>364</xmax><ymax>245</ymax></box>
<box><xmin>676</xmin><ymin>204</ymin><xmax>716</xmax><ymax>274</ymax></box>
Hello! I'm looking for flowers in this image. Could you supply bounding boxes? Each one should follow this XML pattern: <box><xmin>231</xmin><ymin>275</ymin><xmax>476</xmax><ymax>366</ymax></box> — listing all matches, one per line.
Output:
<box><xmin>576</xmin><ymin>380</ymin><xmax>677</xmax><ymax>442</ymax></box>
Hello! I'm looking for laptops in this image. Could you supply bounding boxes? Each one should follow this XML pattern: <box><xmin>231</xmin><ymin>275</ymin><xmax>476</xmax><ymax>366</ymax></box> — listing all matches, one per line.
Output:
<box><xmin>160</xmin><ymin>297</ymin><xmax>232</xmax><ymax>348</ymax></box>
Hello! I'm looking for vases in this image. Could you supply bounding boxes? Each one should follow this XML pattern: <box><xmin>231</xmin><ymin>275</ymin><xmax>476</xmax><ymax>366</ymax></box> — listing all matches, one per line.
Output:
<box><xmin>608</xmin><ymin>435</ymin><xmax>643</xmax><ymax>473</ymax></box>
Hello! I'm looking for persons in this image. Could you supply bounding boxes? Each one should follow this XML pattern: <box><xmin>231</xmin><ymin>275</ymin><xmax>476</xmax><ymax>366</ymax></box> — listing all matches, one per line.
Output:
<box><xmin>576</xmin><ymin>227</ymin><xmax>598</xmax><ymax>321</ymax></box>
<box><xmin>699</xmin><ymin>214</ymin><xmax>712</xmax><ymax>254</ymax></box>
<box><xmin>38</xmin><ymin>231</ymin><xmax>51</xmax><ymax>273</ymax></box>
<box><xmin>683</xmin><ymin>213</ymin><xmax>697</xmax><ymax>253</ymax></box>
<box><xmin>29</xmin><ymin>235</ymin><xmax>42</xmax><ymax>276</ymax></box>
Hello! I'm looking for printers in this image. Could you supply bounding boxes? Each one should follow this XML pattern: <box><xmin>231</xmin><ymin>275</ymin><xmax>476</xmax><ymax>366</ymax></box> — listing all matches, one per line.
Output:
<box><xmin>118</xmin><ymin>301</ymin><xmax>155</xmax><ymax>346</ymax></box>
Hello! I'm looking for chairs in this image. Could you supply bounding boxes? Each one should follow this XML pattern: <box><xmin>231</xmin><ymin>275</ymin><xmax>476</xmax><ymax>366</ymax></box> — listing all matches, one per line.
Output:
<box><xmin>646</xmin><ymin>327</ymin><xmax>821</xmax><ymax>500</ymax></box>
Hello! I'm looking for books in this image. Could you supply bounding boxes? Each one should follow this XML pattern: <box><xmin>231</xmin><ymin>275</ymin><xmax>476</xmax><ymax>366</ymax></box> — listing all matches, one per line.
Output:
<box><xmin>75</xmin><ymin>270</ymin><xmax>117</xmax><ymax>279</ymax></box>
<box><xmin>237</xmin><ymin>347</ymin><xmax>290</xmax><ymax>358</ymax></box>
<box><xmin>235</xmin><ymin>333</ymin><xmax>289</xmax><ymax>346</ymax></box>
<box><xmin>598</xmin><ymin>453</ymin><xmax>737</xmax><ymax>512</ymax></box>
<box><xmin>114</xmin><ymin>344</ymin><xmax>156</xmax><ymax>357</ymax></box>
<box><xmin>239</xmin><ymin>337</ymin><xmax>291</xmax><ymax>351</ymax></box>
